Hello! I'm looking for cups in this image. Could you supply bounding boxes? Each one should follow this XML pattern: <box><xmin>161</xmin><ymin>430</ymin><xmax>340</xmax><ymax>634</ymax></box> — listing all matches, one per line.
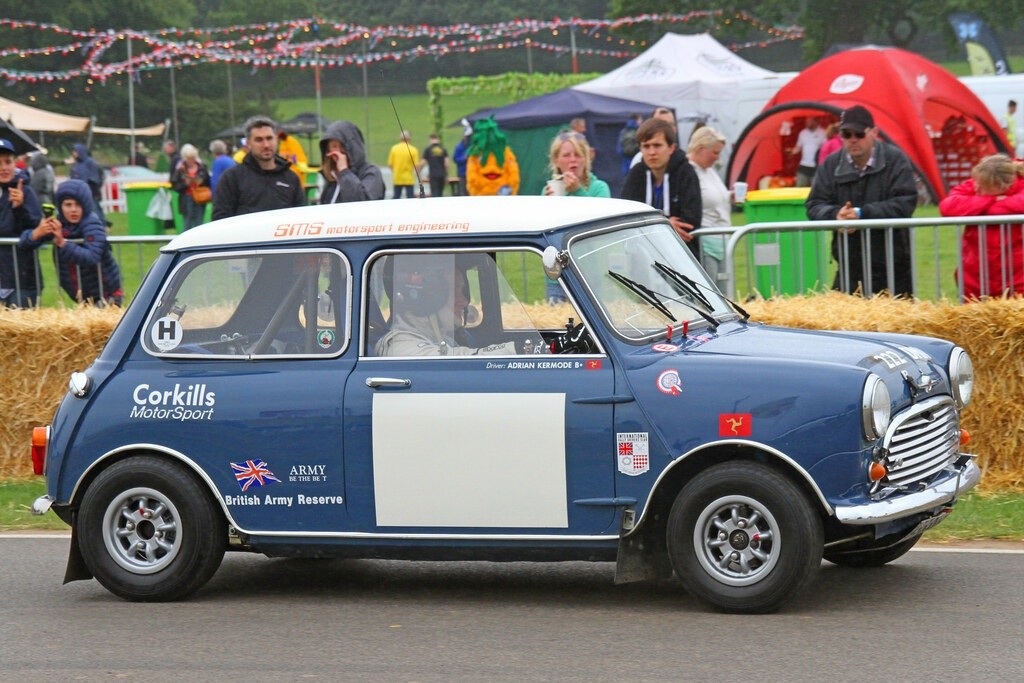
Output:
<box><xmin>547</xmin><ymin>180</ymin><xmax>565</xmax><ymax>196</ymax></box>
<box><xmin>733</xmin><ymin>182</ymin><xmax>748</xmax><ymax>203</ymax></box>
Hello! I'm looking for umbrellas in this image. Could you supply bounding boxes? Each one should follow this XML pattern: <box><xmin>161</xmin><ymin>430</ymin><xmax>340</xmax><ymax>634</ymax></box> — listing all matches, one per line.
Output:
<box><xmin>206</xmin><ymin>112</ymin><xmax>339</xmax><ymax>169</ymax></box>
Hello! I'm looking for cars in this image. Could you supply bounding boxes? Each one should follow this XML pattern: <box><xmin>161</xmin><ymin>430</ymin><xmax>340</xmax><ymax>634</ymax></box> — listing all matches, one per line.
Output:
<box><xmin>28</xmin><ymin>196</ymin><xmax>983</xmax><ymax>617</ymax></box>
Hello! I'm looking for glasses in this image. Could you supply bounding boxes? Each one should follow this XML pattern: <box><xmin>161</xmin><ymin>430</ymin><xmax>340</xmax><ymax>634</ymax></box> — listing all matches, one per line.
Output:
<box><xmin>841</xmin><ymin>131</ymin><xmax>866</xmax><ymax>139</ymax></box>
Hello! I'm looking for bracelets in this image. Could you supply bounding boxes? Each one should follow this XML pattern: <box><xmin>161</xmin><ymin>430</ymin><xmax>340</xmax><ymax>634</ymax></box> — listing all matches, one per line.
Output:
<box><xmin>853</xmin><ymin>206</ymin><xmax>862</xmax><ymax>216</ymax></box>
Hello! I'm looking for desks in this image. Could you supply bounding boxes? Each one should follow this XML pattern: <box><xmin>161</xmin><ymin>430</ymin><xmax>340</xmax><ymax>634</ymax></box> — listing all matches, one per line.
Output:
<box><xmin>422</xmin><ymin>176</ymin><xmax>461</xmax><ymax>196</ymax></box>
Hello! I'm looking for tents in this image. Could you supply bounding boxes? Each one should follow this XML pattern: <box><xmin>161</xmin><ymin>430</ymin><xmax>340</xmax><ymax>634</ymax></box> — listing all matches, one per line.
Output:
<box><xmin>0</xmin><ymin>95</ymin><xmax>166</xmax><ymax>167</ymax></box>
<box><xmin>465</xmin><ymin>32</ymin><xmax>1024</xmax><ymax>204</ymax></box>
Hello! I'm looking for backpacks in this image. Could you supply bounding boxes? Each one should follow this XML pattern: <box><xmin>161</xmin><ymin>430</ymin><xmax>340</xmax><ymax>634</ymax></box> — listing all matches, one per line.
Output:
<box><xmin>621</xmin><ymin>129</ymin><xmax>639</xmax><ymax>154</ymax></box>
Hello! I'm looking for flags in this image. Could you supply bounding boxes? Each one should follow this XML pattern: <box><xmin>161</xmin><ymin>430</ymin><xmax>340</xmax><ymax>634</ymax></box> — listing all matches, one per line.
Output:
<box><xmin>231</xmin><ymin>459</ymin><xmax>281</xmax><ymax>491</ymax></box>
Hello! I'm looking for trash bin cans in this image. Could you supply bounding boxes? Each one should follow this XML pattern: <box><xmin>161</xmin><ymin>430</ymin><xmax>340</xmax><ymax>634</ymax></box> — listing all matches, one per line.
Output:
<box><xmin>307</xmin><ymin>167</ymin><xmax>322</xmax><ymax>206</ymax></box>
<box><xmin>121</xmin><ymin>182</ymin><xmax>166</xmax><ymax>235</ymax></box>
<box><xmin>734</xmin><ymin>187</ymin><xmax>829</xmax><ymax>300</ymax></box>
<box><xmin>166</xmin><ymin>182</ymin><xmax>213</xmax><ymax>234</ymax></box>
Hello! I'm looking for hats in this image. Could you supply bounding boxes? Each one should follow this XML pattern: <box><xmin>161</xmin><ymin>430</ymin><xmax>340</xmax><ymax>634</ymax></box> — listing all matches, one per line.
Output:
<box><xmin>0</xmin><ymin>138</ymin><xmax>15</xmax><ymax>152</ymax></box>
<box><xmin>839</xmin><ymin>106</ymin><xmax>875</xmax><ymax>131</ymax></box>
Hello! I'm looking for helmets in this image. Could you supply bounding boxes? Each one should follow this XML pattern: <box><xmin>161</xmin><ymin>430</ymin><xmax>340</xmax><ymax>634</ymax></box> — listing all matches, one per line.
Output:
<box><xmin>383</xmin><ymin>253</ymin><xmax>476</xmax><ymax>316</ymax></box>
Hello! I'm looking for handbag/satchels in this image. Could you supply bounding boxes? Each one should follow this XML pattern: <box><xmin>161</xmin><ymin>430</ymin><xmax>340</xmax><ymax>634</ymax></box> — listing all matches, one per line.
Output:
<box><xmin>193</xmin><ymin>187</ymin><xmax>212</xmax><ymax>201</ymax></box>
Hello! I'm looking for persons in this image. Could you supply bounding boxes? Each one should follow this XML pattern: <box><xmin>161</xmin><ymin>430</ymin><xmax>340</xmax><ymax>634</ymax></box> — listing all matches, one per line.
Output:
<box><xmin>0</xmin><ymin>117</ymin><xmax>467</xmax><ymax>309</ymax></box>
<box><xmin>370</xmin><ymin>248</ymin><xmax>597</xmax><ymax>355</ymax></box>
<box><xmin>542</xmin><ymin>105</ymin><xmax>1024</xmax><ymax>303</ymax></box>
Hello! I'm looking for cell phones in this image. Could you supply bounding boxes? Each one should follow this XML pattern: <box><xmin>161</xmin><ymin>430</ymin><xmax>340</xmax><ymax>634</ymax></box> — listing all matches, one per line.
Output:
<box><xmin>41</xmin><ymin>203</ymin><xmax>56</xmax><ymax>220</ymax></box>
